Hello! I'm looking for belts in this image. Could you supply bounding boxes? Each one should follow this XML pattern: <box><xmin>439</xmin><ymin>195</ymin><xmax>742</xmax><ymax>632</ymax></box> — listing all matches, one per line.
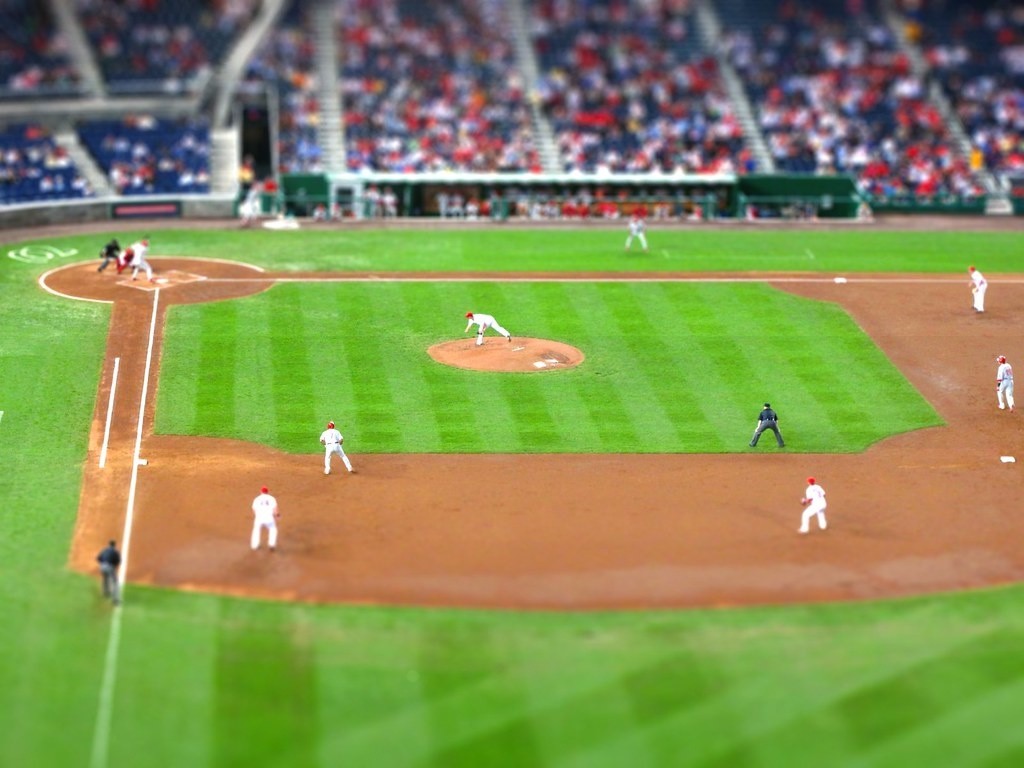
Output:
<box><xmin>328</xmin><ymin>441</ymin><xmax>339</xmax><ymax>445</ymax></box>
<box><xmin>766</xmin><ymin>418</ymin><xmax>775</xmax><ymax>421</ymax></box>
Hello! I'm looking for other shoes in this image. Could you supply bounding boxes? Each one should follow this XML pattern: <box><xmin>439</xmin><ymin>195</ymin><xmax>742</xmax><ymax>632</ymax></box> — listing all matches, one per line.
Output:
<box><xmin>507</xmin><ymin>335</ymin><xmax>511</xmax><ymax>343</ymax></box>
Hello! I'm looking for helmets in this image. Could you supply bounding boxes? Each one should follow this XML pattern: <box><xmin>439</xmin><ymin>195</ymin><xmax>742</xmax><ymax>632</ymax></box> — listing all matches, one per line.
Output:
<box><xmin>328</xmin><ymin>421</ymin><xmax>335</xmax><ymax>429</ymax></box>
<box><xmin>999</xmin><ymin>356</ymin><xmax>1005</xmax><ymax>364</ymax></box>
<box><xmin>807</xmin><ymin>477</ymin><xmax>816</xmax><ymax>484</ymax></box>
<box><xmin>466</xmin><ymin>312</ymin><xmax>473</xmax><ymax>317</ymax></box>
<box><xmin>261</xmin><ymin>487</ymin><xmax>269</xmax><ymax>494</ymax></box>
<box><xmin>969</xmin><ymin>266</ymin><xmax>975</xmax><ymax>272</ymax></box>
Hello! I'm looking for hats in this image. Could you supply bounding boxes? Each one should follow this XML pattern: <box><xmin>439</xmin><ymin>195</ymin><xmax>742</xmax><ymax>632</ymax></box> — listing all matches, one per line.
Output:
<box><xmin>109</xmin><ymin>541</ymin><xmax>116</xmax><ymax>546</ymax></box>
<box><xmin>764</xmin><ymin>402</ymin><xmax>770</xmax><ymax>408</ymax></box>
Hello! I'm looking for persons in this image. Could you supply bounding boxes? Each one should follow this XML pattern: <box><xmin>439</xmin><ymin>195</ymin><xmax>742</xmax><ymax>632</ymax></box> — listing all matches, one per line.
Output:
<box><xmin>995</xmin><ymin>355</ymin><xmax>1016</xmax><ymax>413</ymax></box>
<box><xmin>797</xmin><ymin>477</ymin><xmax>827</xmax><ymax>534</ymax></box>
<box><xmin>464</xmin><ymin>312</ymin><xmax>512</xmax><ymax>346</ymax></box>
<box><xmin>319</xmin><ymin>421</ymin><xmax>356</xmax><ymax>475</ymax></box>
<box><xmin>95</xmin><ymin>540</ymin><xmax>122</xmax><ymax>606</ymax></box>
<box><xmin>748</xmin><ymin>403</ymin><xmax>786</xmax><ymax>449</ymax></box>
<box><xmin>97</xmin><ymin>238</ymin><xmax>157</xmax><ymax>283</ymax></box>
<box><xmin>250</xmin><ymin>486</ymin><xmax>280</xmax><ymax>550</ymax></box>
<box><xmin>967</xmin><ymin>266</ymin><xmax>988</xmax><ymax>313</ymax></box>
<box><xmin>1</xmin><ymin>0</ymin><xmax>1024</xmax><ymax>228</ymax></box>
<box><xmin>622</xmin><ymin>213</ymin><xmax>648</xmax><ymax>252</ymax></box>
